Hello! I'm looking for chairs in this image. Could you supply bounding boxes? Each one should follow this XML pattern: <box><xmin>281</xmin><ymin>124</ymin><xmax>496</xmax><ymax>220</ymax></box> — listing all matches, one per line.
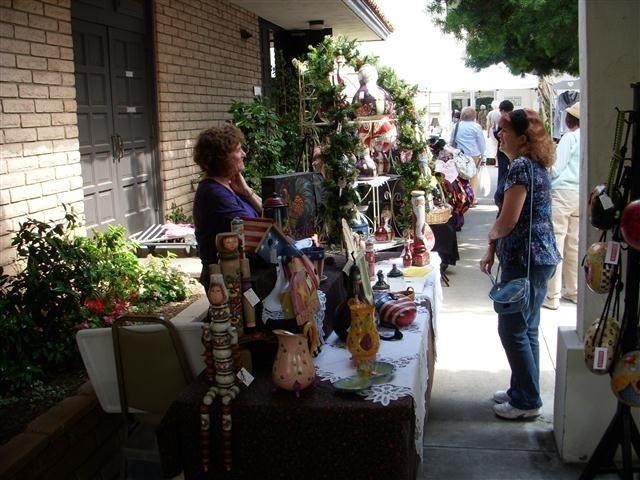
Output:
<box><xmin>112</xmin><ymin>315</ymin><xmax>194</xmax><ymax>479</ymax></box>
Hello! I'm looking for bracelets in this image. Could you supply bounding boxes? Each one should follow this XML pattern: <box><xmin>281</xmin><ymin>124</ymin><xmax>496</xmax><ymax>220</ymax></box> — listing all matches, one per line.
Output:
<box><xmin>248</xmin><ymin>189</ymin><xmax>255</xmax><ymax>201</ymax></box>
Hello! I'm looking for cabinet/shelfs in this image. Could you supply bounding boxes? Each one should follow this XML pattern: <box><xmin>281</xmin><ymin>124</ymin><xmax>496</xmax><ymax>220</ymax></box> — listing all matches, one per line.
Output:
<box><xmin>305</xmin><ymin>116</ymin><xmax>414</xmax><ymax>250</ymax></box>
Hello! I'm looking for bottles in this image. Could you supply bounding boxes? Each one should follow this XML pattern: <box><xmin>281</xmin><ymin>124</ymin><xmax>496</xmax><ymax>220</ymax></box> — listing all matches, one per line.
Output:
<box><xmin>371</xmin><ymin>274</ymin><xmax>390</xmax><ymax>294</ymax></box>
<box><xmin>376</xmin><ymin>214</ymin><xmax>395</xmax><ymax>243</ymax></box>
<box><xmin>403</xmin><ymin>245</ymin><xmax>413</xmax><ymax>268</ymax></box>
<box><xmin>387</xmin><ymin>264</ymin><xmax>404</xmax><ymax>291</ymax></box>
<box><xmin>364</xmin><ymin>240</ymin><xmax>375</xmax><ymax>277</ymax></box>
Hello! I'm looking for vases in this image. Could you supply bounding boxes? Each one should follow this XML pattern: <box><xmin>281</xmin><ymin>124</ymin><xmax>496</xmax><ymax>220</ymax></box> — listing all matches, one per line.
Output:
<box><xmin>346</xmin><ymin>303</ymin><xmax>381</xmax><ymax>362</ymax></box>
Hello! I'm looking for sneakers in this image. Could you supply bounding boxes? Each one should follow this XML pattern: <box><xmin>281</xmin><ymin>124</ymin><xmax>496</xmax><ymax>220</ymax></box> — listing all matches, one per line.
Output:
<box><xmin>493</xmin><ymin>402</ymin><xmax>540</xmax><ymax>419</ymax></box>
<box><xmin>493</xmin><ymin>392</ymin><xmax>513</xmax><ymax>404</ymax></box>
<box><xmin>560</xmin><ymin>287</ymin><xmax>578</xmax><ymax>303</ymax></box>
<box><xmin>541</xmin><ymin>295</ymin><xmax>561</xmax><ymax>309</ymax></box>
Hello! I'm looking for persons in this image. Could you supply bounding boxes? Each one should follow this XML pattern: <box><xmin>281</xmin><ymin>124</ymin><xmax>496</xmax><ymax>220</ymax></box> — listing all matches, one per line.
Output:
<box><xmin>494</xmin><ymin>100</ymin><xmax>515</xmax><ymax>209</ymax></box>
<box><xmin>450</xmin><ymin>107</ymin><xmax>487</xmax><ymax>207</ymax></box>
<box><xmin>193</xmin><ymin>125</ymin><xmax>262</xmax><ymax>299</ymax></box>
<box><xmin>542</xmin><ymin>102</ymin><xmax>581</xmax><ymax>310</ymax></box>
<box><xmin>477</xmin><ymin>104</ymin><xmax>489</xmax><ymax>129</ymax></box>
<box><xmin>480</xmin><ymin>109</ymin><xmax>563</xmax><ymax>421</ymax></box>
<box><xmin>486</xmin><ymin>98</ymin><xmax>500</xmax><ymax>137</ymax></box>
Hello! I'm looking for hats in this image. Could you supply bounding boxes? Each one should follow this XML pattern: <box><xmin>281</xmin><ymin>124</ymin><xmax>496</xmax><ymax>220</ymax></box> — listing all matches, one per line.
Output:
<box><xmin>565</xmin><ymin>101</ymin><xmax>579</xmax><ymax>119</ymax></box>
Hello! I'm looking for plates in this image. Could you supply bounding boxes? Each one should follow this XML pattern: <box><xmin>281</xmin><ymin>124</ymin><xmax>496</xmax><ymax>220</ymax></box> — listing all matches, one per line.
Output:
<box><xmin>357</xmin><ymin>361</ymin><xmax>394</xmax><ymax>378</ymax></box>
<box><xmin>332</xmin><ymin>375</ymin><xmax>372</xmax><ymax>390</ymax></box>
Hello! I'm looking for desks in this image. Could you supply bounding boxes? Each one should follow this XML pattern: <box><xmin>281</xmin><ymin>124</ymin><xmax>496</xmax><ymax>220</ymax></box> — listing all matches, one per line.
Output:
<box><xmin>233</xmin><ymin>249</ymin><xmax>443</xmax><ymax>480</ymax></box>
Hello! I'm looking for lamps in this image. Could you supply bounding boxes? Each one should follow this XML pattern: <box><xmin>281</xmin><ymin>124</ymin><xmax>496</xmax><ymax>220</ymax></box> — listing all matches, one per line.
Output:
<box><xmin>308</xmin><ymin>20</ymin><xmax>324</xmax><ymax>30</ymax></box>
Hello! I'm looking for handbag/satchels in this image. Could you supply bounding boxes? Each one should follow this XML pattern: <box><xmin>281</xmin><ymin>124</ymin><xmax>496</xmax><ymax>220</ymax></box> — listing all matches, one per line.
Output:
<box><xmin>589</xmin><ymin>182</ymin><xmax>624</xmax><ymax>230</ymax></box>
<box><xmin>580</xmin><ymin>241</ymin><xmax>616</xmax><ymax>294</ymax></box>
<box><xmin>374</xmin><ymin>291</ymin><xmax>416</xmax><ymax>339</ymax></box>
<box><xmin>584</xmin><ymin>316</ymin><xmax>621</xmax><ymax>375</ymax></box>
<box><xmin>621</xmin><ymin>197</ymin><xmax>640</xmax><ymax>251</ymax></box>
<box><xmin>455</xmin><ymin>150</ymin><xmax>477</xmax><ymax>180</ymax></box>
<box><xmin>610</xmin><ymin>349</ymin><xmax>640</xmax><ymax>408</ymax></box>
<box><xmin>489</xmin><ymin>277</ymin><xmax>531</xmax><ymax>316</ymax></box>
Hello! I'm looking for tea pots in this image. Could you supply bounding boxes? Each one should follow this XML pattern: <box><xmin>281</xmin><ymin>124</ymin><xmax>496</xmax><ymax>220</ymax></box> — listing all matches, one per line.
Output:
<box><xmin>346</xmin><ymin>297</ymin><xmax>381</xmax><ymax>378</ymax></box>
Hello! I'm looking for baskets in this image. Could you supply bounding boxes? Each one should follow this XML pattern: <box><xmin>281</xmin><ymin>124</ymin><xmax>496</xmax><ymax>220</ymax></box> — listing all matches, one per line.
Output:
<box><xmin>426</xmin><ymin>204</ymin><xmax>452</xmax><ymax>226</ymax></box>
<box><xmin>398</xmin><ymin>286</ymin><xmax>415</xmax><ymax>301</ymax></box>
